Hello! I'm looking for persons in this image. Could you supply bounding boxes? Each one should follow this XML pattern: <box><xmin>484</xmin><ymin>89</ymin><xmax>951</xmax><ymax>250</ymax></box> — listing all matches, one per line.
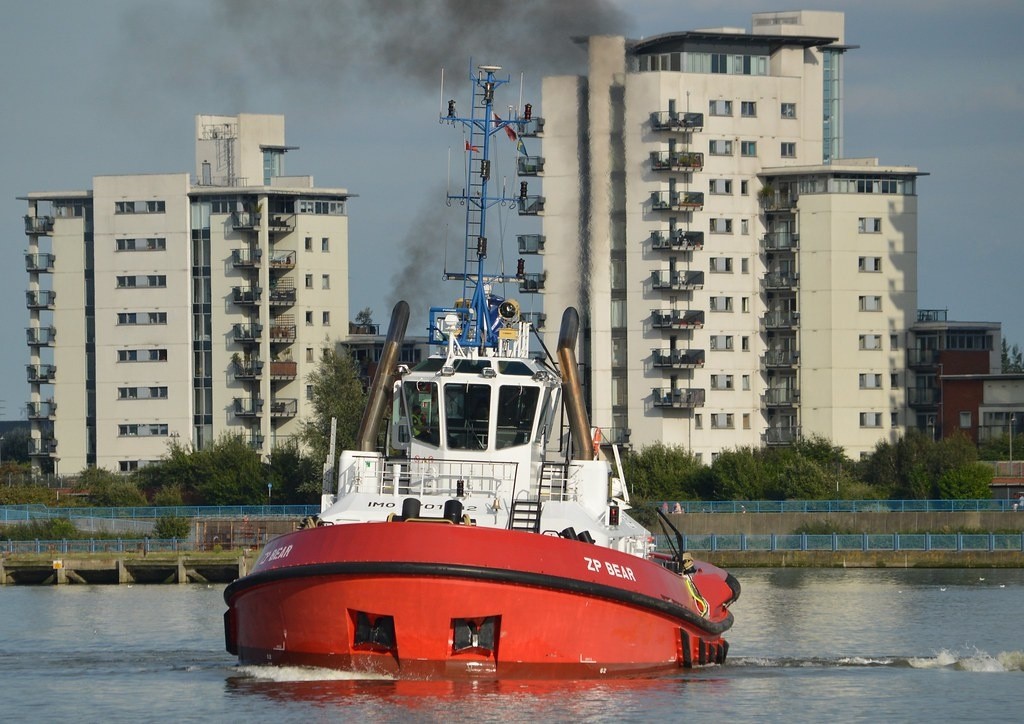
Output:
<box><xmin>410</xmin><ymin>405</ymin><xmax>457</xmax><ymax>447</ymax></box>
<box><xmin>742</xmin><ymin>506</ymin><xmax>746</xmax><ymax>513</ymax></box>
<box><xmin>658</xmin><ymin>502</ymin><xmax>685</xmax><ymax>514</ymax></box>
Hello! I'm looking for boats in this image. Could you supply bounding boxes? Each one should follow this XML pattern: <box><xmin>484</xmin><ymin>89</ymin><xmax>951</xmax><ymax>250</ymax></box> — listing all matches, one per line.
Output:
<box><xmin>219</xmin><ymin>58</ymin><xmax>741</xmax><ymax>662</ymax></box>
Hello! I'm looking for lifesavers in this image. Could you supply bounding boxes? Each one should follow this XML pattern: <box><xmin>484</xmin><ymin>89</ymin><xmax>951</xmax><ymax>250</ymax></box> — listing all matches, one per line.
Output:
<box><xmin>593</xmin><ymin>426</ymin><xmax>601</xmax><ymax>455</ymax></box>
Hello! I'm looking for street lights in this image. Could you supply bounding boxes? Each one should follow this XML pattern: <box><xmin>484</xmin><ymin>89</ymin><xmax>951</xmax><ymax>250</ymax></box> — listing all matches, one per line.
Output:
<box><xmin>1009</xmin><ymin>417</ymin><xmax>1015</xmax><ymax>477</ymax></box>
